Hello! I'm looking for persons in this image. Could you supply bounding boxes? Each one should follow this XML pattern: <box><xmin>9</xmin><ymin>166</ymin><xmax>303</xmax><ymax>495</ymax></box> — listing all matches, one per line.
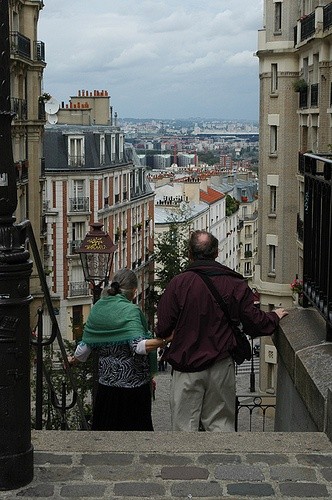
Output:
<box><xmin>152</xmin><ymin>230</ymin><xmax>289</xmax><ymax>434</ymax></box>
<box><xmin>71</xmin><ymin>267</ymin><xmax>177</xmax><ymax>433</ymax></box>
<box><xmin>157</xmin><ymin>343</ymin><xmax>169</xmax><ymax>372</ymax></box>
<box><xmin>152</xmin><ymin>376</ymin><xmax>156</xmax><ymax>401</ymax></box>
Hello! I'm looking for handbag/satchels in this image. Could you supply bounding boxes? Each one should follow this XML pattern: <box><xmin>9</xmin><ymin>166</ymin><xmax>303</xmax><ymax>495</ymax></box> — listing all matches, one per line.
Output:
<box><xmin>230</xmin><ymin>324</ymin><xmax>252</xmax><ymax>364</ymax></box>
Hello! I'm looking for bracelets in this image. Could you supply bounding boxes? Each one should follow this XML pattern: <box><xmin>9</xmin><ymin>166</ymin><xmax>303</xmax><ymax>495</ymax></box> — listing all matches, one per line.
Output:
<box><xmin>157</xmin><ymin>337</ymin><xmax>169</xmax><ymax>346</ymax></box>
